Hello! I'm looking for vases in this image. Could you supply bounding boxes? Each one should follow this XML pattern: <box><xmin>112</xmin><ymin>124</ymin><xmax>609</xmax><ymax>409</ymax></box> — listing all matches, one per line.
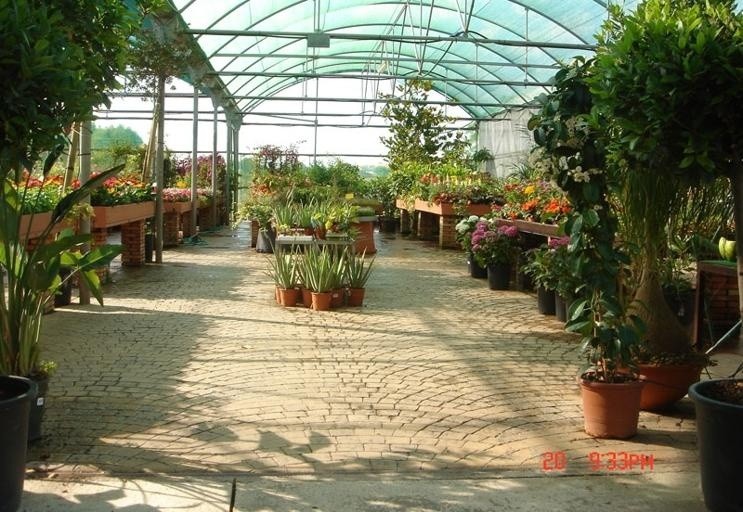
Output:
<box><xmin>537</xmin><ymin>287</ymin><xmax>555</xmax><ymax>315</ymax></box>
<box><xmin>577</xmin><ymin>382</ymin><xmax>639</xmax><ymax>440</ymax></box>
<box><xmin>555</xmin><ymin>291</ymin><xmax>569</xmax><ymax>321</ymax></box>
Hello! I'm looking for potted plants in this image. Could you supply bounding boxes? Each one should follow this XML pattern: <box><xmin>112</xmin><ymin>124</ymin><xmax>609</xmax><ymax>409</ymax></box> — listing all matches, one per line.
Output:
<box><xmin>639</xmin><ymin>1</ymin><xmax>742</xmax><ymax>510</ymax></box>
<box><xmin>0</xmin><ymin>156</ymin><xmax>127</xmax><ymax>450</ymax></box>
<box><xmin>421</xmin><ymin>162</ymin><xmax>499</xmax><ymax>204</ymax></box>
<box><xmin>228</xmin><ymin>176</ymin><xmax>379</xmax><ymax>311</ymax></box>
<box><xmin>577</xmin><ymin>43</ymin><xmax>719</xmax><ymax>412</ymax></box>
<box><xmin>0</xmin><ymin>0</ymin><xmax>190</xmax><ymax>512</ymax></box>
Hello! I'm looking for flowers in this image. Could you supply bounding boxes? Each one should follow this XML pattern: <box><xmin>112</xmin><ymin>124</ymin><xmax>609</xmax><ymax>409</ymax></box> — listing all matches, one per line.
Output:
<box><xmin>456</xmin><ymin>176</ymin><xmax>577</xmax><ymax>296</ymax></box>
<box><xmin>7</xmin><ymin>152</ymin><xmax>227</xmax><ymax>215</ymax></box>
